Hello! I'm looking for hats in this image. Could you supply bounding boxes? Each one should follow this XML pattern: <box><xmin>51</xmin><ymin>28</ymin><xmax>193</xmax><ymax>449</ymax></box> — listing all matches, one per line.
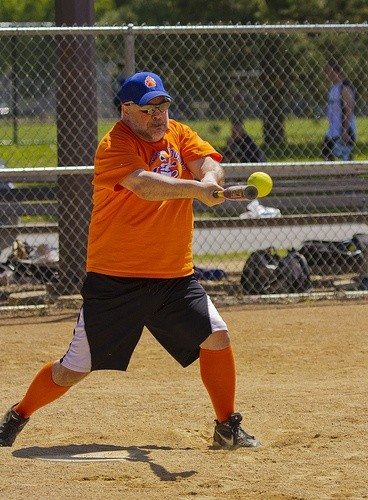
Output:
<box><xmin>117</xmin><ymin>72</ymin><xmax>173</xmax><ymax>107</ymax></box>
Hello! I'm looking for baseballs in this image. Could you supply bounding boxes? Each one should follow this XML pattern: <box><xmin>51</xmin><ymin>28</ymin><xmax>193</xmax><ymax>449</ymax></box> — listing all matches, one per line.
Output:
<box><xmin>248</xmin><ymin>171</ymin><xmax>273</xmax><ymax>198</ymax></box>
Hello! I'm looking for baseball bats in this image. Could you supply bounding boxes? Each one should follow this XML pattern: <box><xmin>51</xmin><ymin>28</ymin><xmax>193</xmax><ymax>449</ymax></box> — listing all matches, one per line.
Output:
<box><xmin>214</xmin><ymin>185</ymin><xmax>259</xmax><ymax>201</ymax></box>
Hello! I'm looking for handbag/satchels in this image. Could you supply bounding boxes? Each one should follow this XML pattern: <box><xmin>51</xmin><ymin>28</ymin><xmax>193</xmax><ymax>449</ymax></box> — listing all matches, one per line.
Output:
<box><xmin>240</xmin><ymin>246</ymin><xmax>312</xmax><ymax>295</ymax></box>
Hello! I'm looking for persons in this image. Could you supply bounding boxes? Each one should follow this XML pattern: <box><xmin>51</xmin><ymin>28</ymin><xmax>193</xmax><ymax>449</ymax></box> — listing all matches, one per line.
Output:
<box><xmin>322</xmin><ymin>58</ymin><xmax>357</xmax><ymax>161</ymax></box>
<box><xmin>220</xmin><ymin>117</ymin><xmax>265</xmax><ymax>163</ymax></box>
<box><xmin>112</xmin><ymin>63</ymin><xmax>127</xmax><ymax>118</ymax></box>
<box><xmin>0</xmin><ymin>72</ymin><xmax>262</xmax><ymax>449</ymax></box>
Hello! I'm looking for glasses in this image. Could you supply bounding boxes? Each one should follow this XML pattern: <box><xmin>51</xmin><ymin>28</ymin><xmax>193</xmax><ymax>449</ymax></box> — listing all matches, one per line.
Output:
<box><xmin>129</xmin><ymin>102</ymin><xmax>171</xmax><ymax>114</ymax></box>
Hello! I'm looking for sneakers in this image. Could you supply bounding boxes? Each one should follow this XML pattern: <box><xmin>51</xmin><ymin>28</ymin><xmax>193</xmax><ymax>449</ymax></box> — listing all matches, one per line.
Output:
<box><xmin>0</xmin><ymin>402</ymin><xmax>30</xmax><ymax>447</ymax></box>
<box><xmin>213</xmin><ymin>412</ymin><xmax>261</xmax><ymax>447</ymax></box>
